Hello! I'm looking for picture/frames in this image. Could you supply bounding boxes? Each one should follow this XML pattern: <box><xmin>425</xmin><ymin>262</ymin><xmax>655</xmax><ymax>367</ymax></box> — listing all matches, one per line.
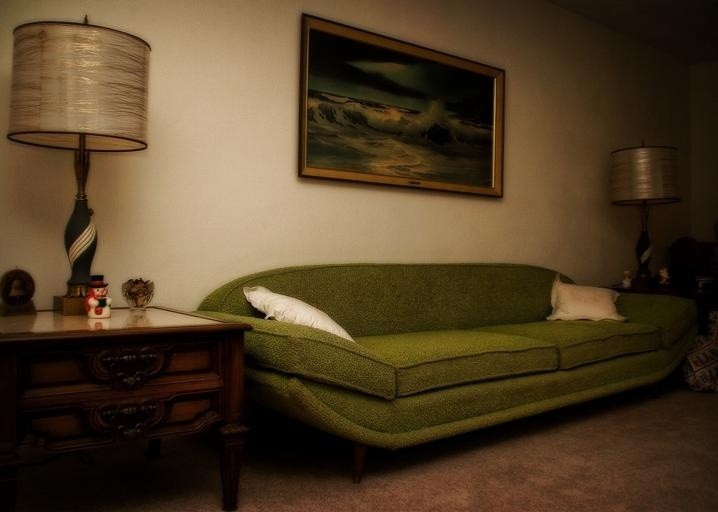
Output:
<box><xmin>298</xmin><ymin>13</ymin><xmax>506</xmax><ymax>199</ymax></box>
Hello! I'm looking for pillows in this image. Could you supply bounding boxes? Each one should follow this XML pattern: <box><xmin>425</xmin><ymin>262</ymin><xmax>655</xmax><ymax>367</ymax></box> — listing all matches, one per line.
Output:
<box><xmin>545</xmin><ymin>271</ymin><xmax>632</xmax><ymax>323</ymax></box>
<box><xmin>243</xmin><ymin>287</ymin><xmax>356</xmax><ymax>348</ymax></box>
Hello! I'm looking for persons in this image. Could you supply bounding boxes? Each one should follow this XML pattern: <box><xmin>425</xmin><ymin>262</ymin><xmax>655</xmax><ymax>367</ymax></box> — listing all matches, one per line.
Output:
<box><xmin>83</xmin><ymin>275</ymin><xmax>112</xmax><ymax>319</ymax></box>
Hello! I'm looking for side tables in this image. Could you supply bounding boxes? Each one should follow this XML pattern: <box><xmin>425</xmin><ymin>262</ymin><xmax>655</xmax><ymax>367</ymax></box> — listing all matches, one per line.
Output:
<box><xmin>1</xmin><ymin>305</ymin><xmax>253</xmax><ymax>510</ymax></box>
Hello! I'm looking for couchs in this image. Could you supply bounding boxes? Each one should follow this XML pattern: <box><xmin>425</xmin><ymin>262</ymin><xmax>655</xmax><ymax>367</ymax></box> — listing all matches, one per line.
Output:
<box><xmin>197</xmin><ymin>263</ymin><xmax>698</xmax><ymax>484</ymax></box>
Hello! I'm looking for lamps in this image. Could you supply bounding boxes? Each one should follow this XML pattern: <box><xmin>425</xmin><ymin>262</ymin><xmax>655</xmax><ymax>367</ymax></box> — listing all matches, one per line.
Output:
<box><xmin>6</xmin><ymin>14</ymin><xmax>152</xmax><ymax>317</ymax></box>
<box><xmin>610</xmin><ymin>140</ymin><xmax>680</xmax><ymax>279</ymax></box>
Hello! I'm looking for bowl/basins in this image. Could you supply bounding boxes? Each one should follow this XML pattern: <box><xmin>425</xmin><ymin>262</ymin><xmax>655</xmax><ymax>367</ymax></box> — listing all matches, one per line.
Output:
<box><xmin>121</xmin><ymin>284</ymin><xmax>154</xmax><ymax>311</ymax></box>
